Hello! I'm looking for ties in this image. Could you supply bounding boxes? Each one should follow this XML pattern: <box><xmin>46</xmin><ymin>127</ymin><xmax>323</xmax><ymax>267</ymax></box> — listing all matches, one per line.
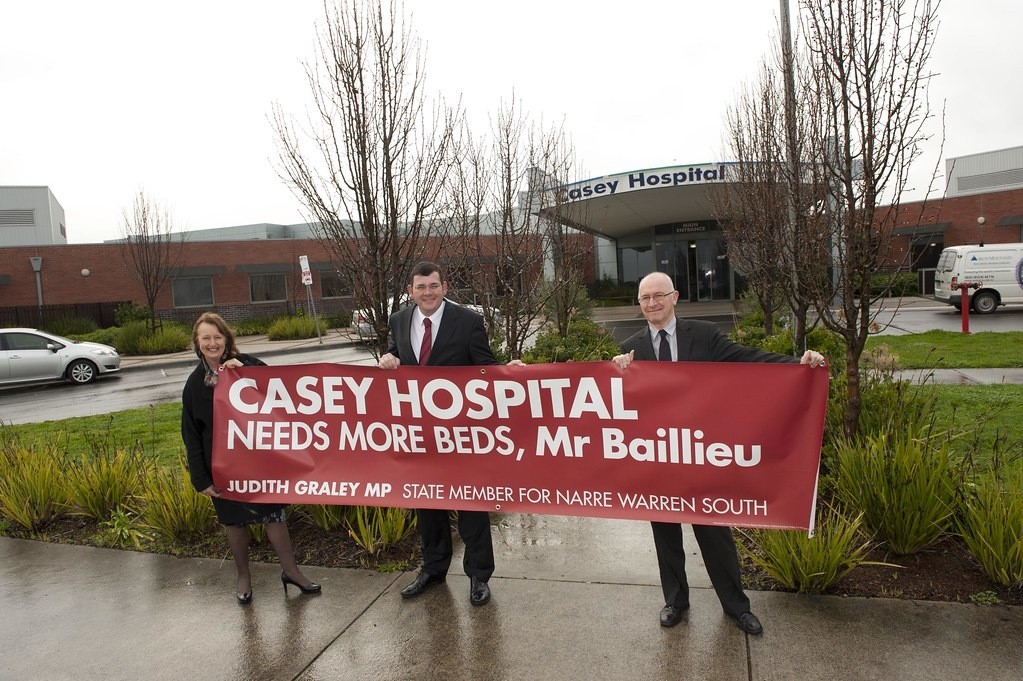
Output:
<box><xmin>419</xmin><ymin>319</ymin><xmax>432</xmax><ymax>366</ymax></box>
<box><xmin>659</xmin><ymin>330</ymin><xmax>672</xmax><ymax>361</ymax></box>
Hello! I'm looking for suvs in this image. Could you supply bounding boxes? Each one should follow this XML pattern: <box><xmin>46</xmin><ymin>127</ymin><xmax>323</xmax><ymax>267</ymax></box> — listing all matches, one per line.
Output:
<box><xmin>934</xmin><ymin>242</ymin><xmax>1023</xmax><ymax>316</ymax></box>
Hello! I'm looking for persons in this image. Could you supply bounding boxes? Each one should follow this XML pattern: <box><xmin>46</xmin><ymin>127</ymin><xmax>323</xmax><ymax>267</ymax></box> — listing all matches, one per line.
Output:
<box><xmin>181</xmin><ymin>312</ymin><xmax>320</xmax><ymax>598</ymax></box>
<box><xmin>375</xmin><ymin>262</ymin><xmax>527</xmax><ymax>604</ymax></box>
<box><xmin>614</xmin><ymin>272</ymin><xmax>824</xmax><ymax>633</ymax></box>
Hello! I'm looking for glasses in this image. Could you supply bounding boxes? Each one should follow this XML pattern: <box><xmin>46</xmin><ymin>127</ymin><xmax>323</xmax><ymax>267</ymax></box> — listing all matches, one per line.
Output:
<box><xmin>412</xmin><ymin>284</ymin><xmax>442</xmax><ymax>291</ymax></box>
<box><xmin>638</xmin><ymin>291</ymin><xmax>674</xmax><ymax>303</ymax></box>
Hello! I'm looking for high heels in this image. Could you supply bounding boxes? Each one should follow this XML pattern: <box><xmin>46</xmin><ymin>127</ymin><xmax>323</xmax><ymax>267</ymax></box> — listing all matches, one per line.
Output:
<box><xmin>236</xmin><ymin>575</ymin><xmax>252</xmax><ymax>603</ymax></box>
<box><xmin>281</xmin><ymin>570</ymin><xmax>321</xmax><ymax>594</ymax></box>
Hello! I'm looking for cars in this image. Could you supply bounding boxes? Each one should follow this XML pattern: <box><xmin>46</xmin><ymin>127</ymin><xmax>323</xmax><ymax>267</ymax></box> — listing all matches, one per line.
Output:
<box><xmin>348</xmin><ymin>294</ymin><xmax>504</xmax><ymax>338</ymax></box>
<box><xmin>0</xmin><ymin>327</ymin><xmax>122</xmax><ymax>391</ymax></box>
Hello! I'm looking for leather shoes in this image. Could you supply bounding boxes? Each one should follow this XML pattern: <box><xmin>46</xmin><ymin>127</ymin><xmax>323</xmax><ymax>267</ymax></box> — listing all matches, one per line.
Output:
<box><xmin>732</xmin><ymin>611</ymin><xmax>763</xmax><ymax>634</ymax></box>
<box><xmin>401</xmin><ymin>571</ymin><xmax>446</xmax><ymax>597</ymax></box>
<box><xmin>660</xmin><ymin>603</ymin><xmax>689</xmax><ymax>625</ymax></box>
<box><xmin>470</xmin><ymin>575</ymin><xmax>490</xmax><ymax>604</ymax></box>
